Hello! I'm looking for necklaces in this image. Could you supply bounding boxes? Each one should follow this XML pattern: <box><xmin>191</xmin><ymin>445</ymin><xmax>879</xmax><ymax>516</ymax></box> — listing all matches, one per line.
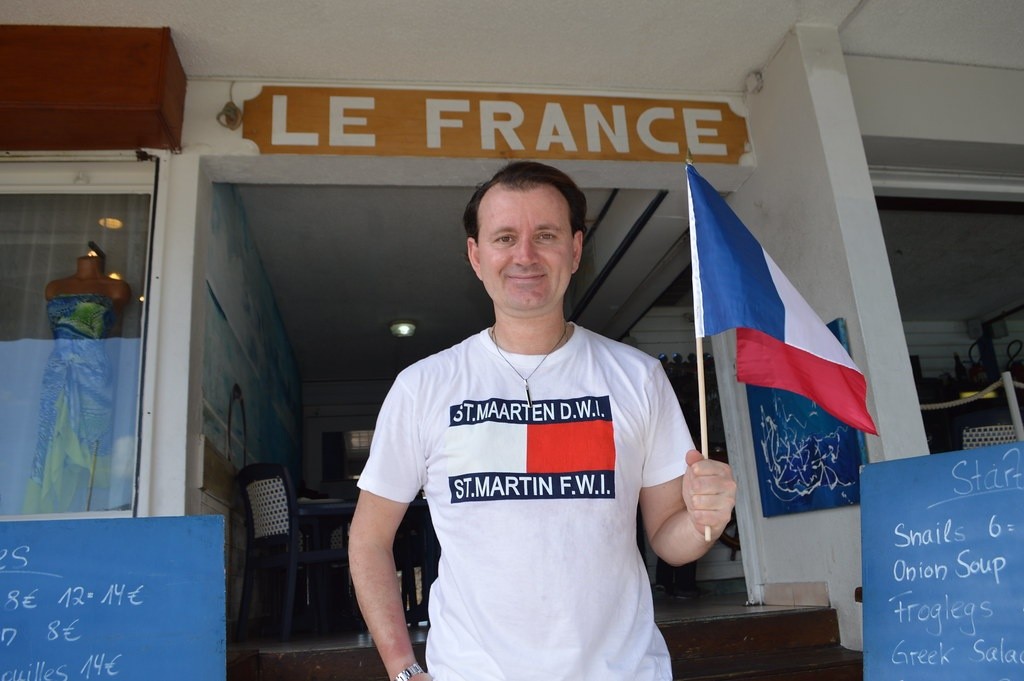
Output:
<box><xmin>492</xmin><ymin>321</ymin><xmax>566</xmax><ymax>408</ymax></box>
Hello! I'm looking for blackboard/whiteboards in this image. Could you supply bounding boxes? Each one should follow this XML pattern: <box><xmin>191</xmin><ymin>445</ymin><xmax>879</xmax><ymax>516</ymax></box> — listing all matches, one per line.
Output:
<box><xmin>1</xmin><ymin>514</ymin><xmax>226</xmax><ymax>681</ymax></box>
<box><xmin>860</xmin><ymin>440</ymin><xmax>1024</xmax><ymax>681</ymax></box>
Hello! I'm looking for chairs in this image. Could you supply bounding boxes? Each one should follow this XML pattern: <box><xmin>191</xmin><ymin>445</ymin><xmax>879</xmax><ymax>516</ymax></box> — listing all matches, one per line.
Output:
<box><xmin>235</xmin><ymin>462</ymin><xmax>359</xmax><ymax>644</ymax></box>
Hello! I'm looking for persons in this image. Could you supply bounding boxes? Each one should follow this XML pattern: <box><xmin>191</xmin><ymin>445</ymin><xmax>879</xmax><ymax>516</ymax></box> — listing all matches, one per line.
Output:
<box><xmin>21</xmin><ymin>255</ymin><xmax>132</xmax><ymax>515</ymax></box>
<box><xmin>347</xmin><ymin>162</ymin><xmax>736</xmax><ymax>681</ymax></box>
<box><xmin>653</xmin><ymin>556</ymin><xmax>710</xmax><ymax>603</ymax></box>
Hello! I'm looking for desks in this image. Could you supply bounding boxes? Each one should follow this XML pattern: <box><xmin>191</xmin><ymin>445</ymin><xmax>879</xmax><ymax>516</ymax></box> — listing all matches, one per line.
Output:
<box><xmin>289</xmin><ymin>499</ymin><xmax>438</xmax><ymax>638</ymax></box>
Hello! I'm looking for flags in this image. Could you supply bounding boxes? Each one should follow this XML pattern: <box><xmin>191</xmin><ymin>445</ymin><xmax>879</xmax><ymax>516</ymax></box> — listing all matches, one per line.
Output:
<box><xmin>685</xmin><ymin>165</ymin><xmax>881</xmax><ymax>438</ymax></box>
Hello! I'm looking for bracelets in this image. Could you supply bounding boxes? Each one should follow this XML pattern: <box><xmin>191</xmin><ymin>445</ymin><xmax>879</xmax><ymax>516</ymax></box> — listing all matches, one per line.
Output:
<box><xmin>393</xmin><ymin>662</ymin><xmax>422</xmax><ymax>681</ymax></box>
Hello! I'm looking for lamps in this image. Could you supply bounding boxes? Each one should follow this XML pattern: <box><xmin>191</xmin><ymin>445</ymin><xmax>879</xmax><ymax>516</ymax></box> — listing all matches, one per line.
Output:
<box><xmin>389</xmin><ymin>320</ymin><xmax>417</xmax><ymax>338</ymax></box>
